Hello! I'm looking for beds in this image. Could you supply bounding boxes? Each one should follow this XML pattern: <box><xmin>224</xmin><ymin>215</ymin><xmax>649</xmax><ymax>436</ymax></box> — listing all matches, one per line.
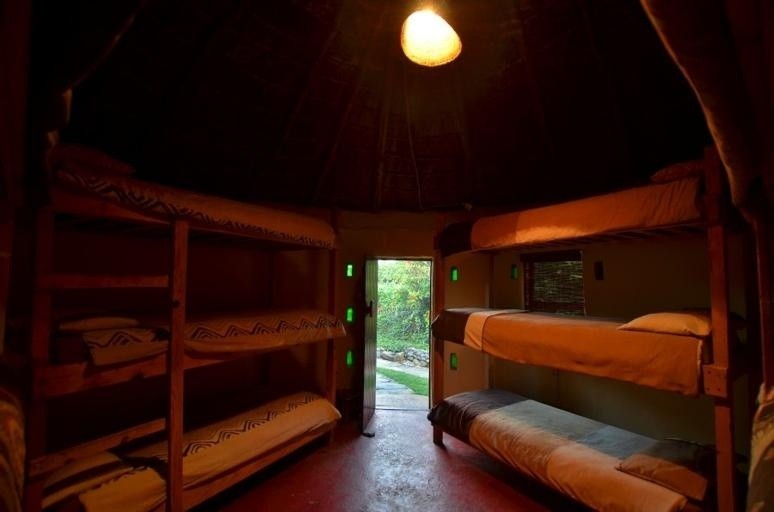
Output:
<box><xmin>1</xmin><ymin>132</ymin><xmax>348</xmax><ymax>512</ymax></box>
<box><xmin>425</xmin><ymin>154</ymin><xmax>773</xmax><ymax>512</ymax></box>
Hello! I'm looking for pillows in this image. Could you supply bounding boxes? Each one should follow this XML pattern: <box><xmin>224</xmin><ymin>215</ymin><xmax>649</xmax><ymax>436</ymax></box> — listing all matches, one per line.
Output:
<box><xmin>3</xmin><ymin>310</ymin><xmax>140</xmax><ymax>334</ymax></box>
<box><xmin>37</xmin><ymin>139</ymin><xmax>134</xmax><ymax>175</ymax></box>
<box><xmin>618</xmin><ymin>306</ymin><xmax>748</xmax><ymax>340</ymax></box>
<box><xmin>40</xmin><ymin>448</ymin><xmax>132</xmax><ymax>504</ymax></box>
